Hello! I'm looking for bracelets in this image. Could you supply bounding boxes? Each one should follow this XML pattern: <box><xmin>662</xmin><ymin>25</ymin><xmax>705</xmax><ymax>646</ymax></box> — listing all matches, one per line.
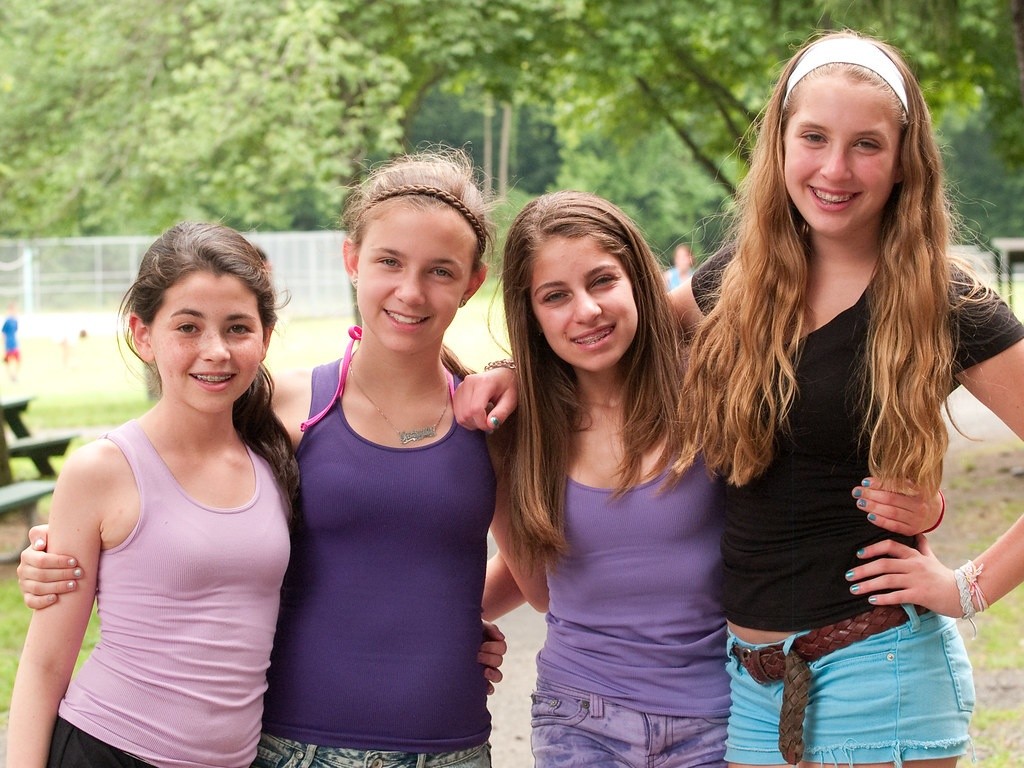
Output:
<box><xmin>954</xmin><ymin>560</ymin><xmax>987</xmax><ymax>638</ymax></box>
<box><xmin>484</xmin><ymin>359</ymin><xmax>514</xmax><ymax>370</ymax></box>
<box><xmin>922</xmin><ymin>490</ymin><xmax>944</xmax><ymax>535</ymax></box>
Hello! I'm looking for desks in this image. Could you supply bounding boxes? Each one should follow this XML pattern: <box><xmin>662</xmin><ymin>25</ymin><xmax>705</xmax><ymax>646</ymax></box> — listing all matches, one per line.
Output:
<box><xmin>0</xmin><ymin>392</ymin><xmax>57</xmax><ymax>482</ymax></box>
<box><xmin>991</xmin><ymin>238</ymin><xmax>1024</xmax><ymax>322</ymax></box>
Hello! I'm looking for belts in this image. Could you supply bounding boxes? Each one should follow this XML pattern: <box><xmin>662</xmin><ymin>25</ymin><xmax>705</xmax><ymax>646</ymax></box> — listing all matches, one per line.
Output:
<box><xmin>731</xmin><ymin>601</ymin><xmax>928</xmax><ymax>766</ymax></box>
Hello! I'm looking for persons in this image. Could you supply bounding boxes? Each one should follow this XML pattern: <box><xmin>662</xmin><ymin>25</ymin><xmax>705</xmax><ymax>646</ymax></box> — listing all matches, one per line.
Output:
<box><xmin>17</xmin><ymin>156</ymin><xmax>549</xmax><ymax>768</ymax></box>
<box><xmin>0</xmin><ymin>303</ymin><xmax>21</xmax><ymax>378</ymax></box>
<box><xmin>451</xmin><ymin>31</ymin><xmax>1024</xmax><ymax>768</ymax></box>
<box><xmin>661</xmin><ymin>245</ymin><xmax>698</xmax><ymax>289</ymax></box>
<box><xmin>6</xmin><ymin>221</ymin><xmax>508</xmax><ymax>768</ymax></box>
<box><xmin>482</xmin><ymin>191</ymin><xmax>944</xmax><ymax>768</ymax></box>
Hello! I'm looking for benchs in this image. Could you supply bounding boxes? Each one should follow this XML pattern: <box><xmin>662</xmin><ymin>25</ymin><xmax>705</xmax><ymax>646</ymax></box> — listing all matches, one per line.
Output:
<box><xmin>0</xmin><ymin>431</ymin><xmax>89</xmax><ymax>567</ymax></box>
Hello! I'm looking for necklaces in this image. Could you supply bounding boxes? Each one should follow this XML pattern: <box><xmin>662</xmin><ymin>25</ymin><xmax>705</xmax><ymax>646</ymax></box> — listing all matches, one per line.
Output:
<box><xmin>349</xmin><ymin>351</ymin><xmax>448</xmax><ymax>443</ymax></box>
